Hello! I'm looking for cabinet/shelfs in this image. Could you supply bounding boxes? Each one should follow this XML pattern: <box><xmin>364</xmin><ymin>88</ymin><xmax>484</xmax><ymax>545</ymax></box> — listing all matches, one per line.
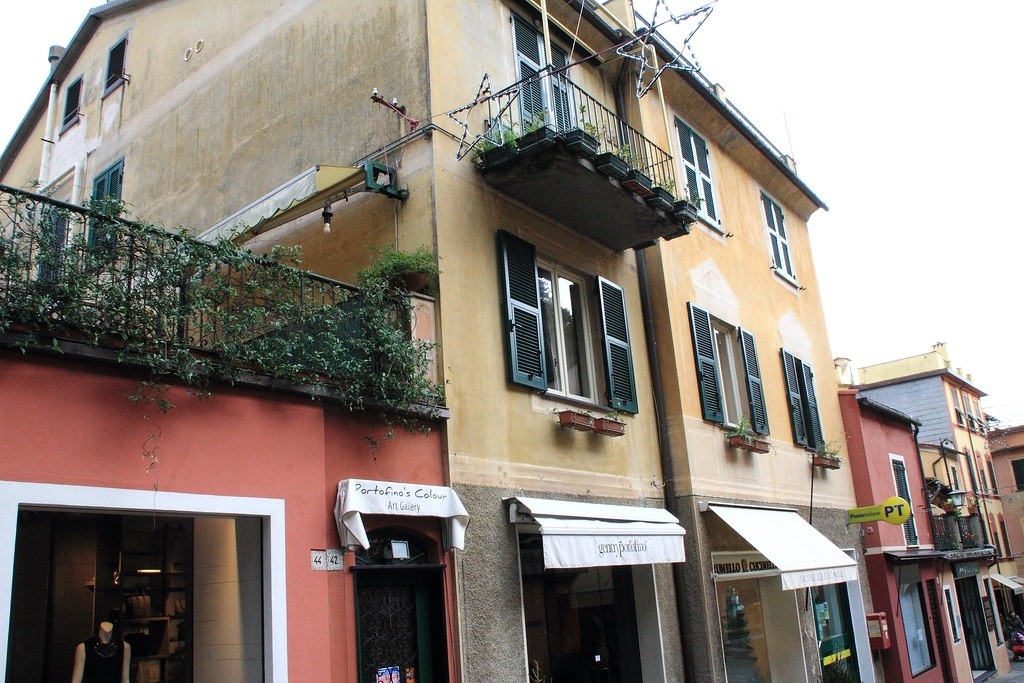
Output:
<box><xmin>114</xmin><ymin>550</ymin><xmax>194</xmax><ymax>683</ymax></box>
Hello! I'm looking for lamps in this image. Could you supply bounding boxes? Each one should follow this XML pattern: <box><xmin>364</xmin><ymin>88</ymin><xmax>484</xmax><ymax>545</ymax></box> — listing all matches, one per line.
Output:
<box><xmin>389</xmin><ymin>539</ymin><xmax>410</xmax><ymax>560</ymax></box>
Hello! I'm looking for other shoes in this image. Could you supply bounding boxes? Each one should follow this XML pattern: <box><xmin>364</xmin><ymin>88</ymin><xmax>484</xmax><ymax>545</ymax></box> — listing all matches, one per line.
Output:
<box><xmin>1014</xmin><ymin>656</ymin><xmax>1018</xmax><ymax>661</ymax></box>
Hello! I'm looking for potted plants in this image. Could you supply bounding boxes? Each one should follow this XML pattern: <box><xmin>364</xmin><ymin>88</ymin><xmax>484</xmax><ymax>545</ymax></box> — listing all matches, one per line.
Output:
<box><xmin>725</xmin><ymin>410</ymin><xmax>754</xmax><ymax>448</ymax></box>
<box><xmin>749</xmin><ymin>434</ymin><xmax>771</xmax><ymax>454</ymax></box>
<box><xmin>477</xmin><ymin>129</ymin><xmax>517</xmax><ymax>167</ymax></box>
<box><xmin>563</xmin><ymin>105</ymin><xmax>607</xmax><ymax>153</ymax></box>
<box><xmin>558</xmin><ymin>408</ymin><xmax>595</xmax><ymax>431</ymax></box>
<box><xmin>812</xmin><ymin>442</ymin><xmax>842</xmax><ymax>469</ymax></box>
<box><xmin>368</xmin><ymin>239</ymin><xmax>440</xmax><ymax>293</ymax></box>
<box><xmin>594</xmin><ymin>409</ymin><xmax>626</xmax><ymax>437</ymax></box>
<box><xmin>623</xmin><ymin>161</ymin><xmax>654</xmax><ymax>194</ymax></box>
<box><xmin>595</xmin><ymin>136</ymin><xmax>630</xmax><ymax>179</ymax></box>
<box><xmin>646</xmin><ymin>178</ymin><xmax>675</xmax><ymax>211</ymax></box>
<box><xmin>516</xmin><ymin>111</ymin><xmax>556</xmax><ymax>153</ymax></box>
<box><xmin>673</xmin><ymin>184</ymin><xmax>703</xmax><ymax>224</ymax></box>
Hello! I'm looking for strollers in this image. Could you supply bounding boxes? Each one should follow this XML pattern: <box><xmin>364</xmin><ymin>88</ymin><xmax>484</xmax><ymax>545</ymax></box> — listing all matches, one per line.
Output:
<box><xmin>1009</xmin><ymin>628</ymin><xmax>1024</xmax><ymax>662</ymax></box>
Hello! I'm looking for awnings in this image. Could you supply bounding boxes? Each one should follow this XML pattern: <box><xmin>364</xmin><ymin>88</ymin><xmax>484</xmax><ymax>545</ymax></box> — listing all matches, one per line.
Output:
<box><xmin>502</xmin><ymin>495</ymin><xmax>688</xmax><ymax>569</ymax></box>
<box><xmin>990</xmin><ymin>573</ymin><xmax>1024</xmax><ymax>595</ymax></box>
<box><xmin>194</xmin><ymin>167</ymin><xmax>362</xmax><ymax>246</ymax></box>
<box><xmin>698</xmin><ymin>499</ymin><xmax>859</xmax><ymax>592</ymax></box>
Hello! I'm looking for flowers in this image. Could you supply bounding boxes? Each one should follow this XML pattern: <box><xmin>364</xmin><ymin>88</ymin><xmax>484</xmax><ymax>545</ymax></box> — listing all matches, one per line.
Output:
<box><xmin>944</xmin><ymin>497</ymin><xmax>980</xmax><ymax>546</ymax></box>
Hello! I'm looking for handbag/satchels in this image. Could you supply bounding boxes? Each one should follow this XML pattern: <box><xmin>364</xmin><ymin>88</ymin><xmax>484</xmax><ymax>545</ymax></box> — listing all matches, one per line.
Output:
<box><xmin>1012</xmin><ymin>632</ymin><xmax>1024</xmax><ymax>645</ymax></box>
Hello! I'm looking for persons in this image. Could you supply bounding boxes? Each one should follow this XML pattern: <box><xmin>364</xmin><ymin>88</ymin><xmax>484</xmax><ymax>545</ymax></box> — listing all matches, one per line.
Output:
<box><xmin>71</xmin><ymin>621</ymin><xmax>131</xmax><ymax>683</ymax></box>
<box><xmin>1004</xmin><ymin>609</ymin><xmax>1024</xmax><ymax>662</ymax></box>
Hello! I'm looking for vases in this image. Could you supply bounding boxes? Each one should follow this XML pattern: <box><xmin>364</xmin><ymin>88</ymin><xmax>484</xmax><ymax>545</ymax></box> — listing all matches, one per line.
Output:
<box><xmin>944</xmin><ymin>504</ymin><xmax>953</xmax><ymax>512</ymax></box>
<box><xmin>968</xmin><ymin>506</ymin><xmax>978</xmax><ymax>514</ymax></box>
